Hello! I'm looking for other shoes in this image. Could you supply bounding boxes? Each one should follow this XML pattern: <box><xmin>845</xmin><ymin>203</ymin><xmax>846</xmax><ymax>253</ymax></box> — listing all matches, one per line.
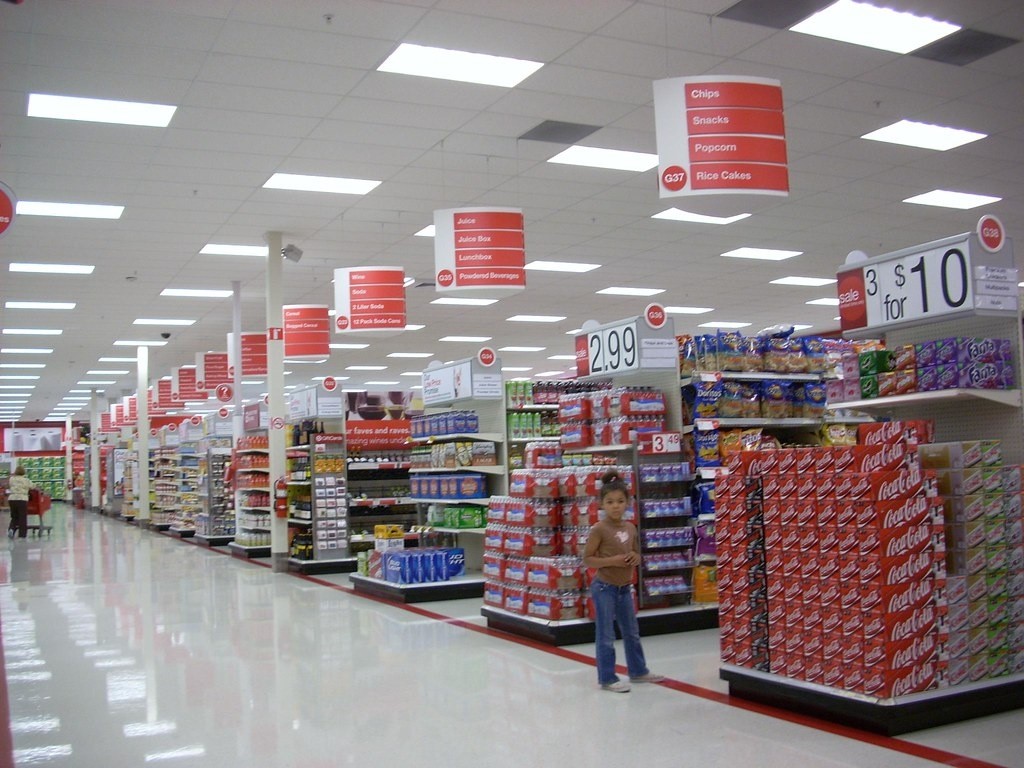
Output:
<box><xmin>629</xmin><ymin>672</ymin><xmax>665</xmax><ymax>683</ymax></box>
<box><xmin>601</xmin><ymin>683</ymin><xmax>631</xmax><ymax>692</ymax></box>
<box><xmin>18</xmin><ymin>537</ymin><xmax>27</xmax><ymax>541</ymax></box>
<box><xmin>8</xmin><ymin>528</ymin><xmax>14</xmax><ymax>539</ymax></box>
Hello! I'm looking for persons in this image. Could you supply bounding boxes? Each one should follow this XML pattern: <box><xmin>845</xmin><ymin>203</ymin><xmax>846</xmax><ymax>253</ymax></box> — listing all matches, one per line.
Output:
<box><xmin>582</xmin><ymin>470</ymin><xmax>665</xmax><ymax>693</ymax></box>
<box><xmin>8</xmin><ymin>466</ymin><xmax>35</xmax><ymax>542</ymax></box>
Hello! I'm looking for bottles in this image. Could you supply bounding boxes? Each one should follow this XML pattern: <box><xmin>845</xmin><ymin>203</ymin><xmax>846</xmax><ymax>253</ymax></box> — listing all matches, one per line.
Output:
<box><xmin>287</xmin><ymin>451</ymin><xmax>311</xmax><ymax>481</ymax></box>
<box><xmin>291</xmin><ymin>534</ymin><xmax>313</xmax><ymax>560</ymax></box>
<box><xmin>410</xmin><ymin>410</ymin><xmax>479</xmax><ymax>438</ymax></box>
<box><xmin>483</xmin><ymin>521</ymin><xmax>640</xmax><ymax>620</ymax></box>
<box><xmin>410</xmin><ymin>444</ymin><xmax>431</xmax><ymax>467</ymax></box>
<box><xmin>237</xmin><ymin>435</ymin><xmax>269</xmax><ymax>451</ymax></box>
<box><xmin>508</xmin><ymin>465</ymin><xmax>638</xmax><ymax>499</ymax></box>
<box><xmin>289</xmin><ymin>495</ymin><xmax>312</xmax><ymax>520</ymax></box>
<box><xmin>238</xmin><ymin>510</ymin><xmax>271</xmax><ymax>526</ymax></box>
<box><xmin>234</xmin><ymin>531</ymin><xmax>272</xmax><ymax>546</ymax></box>
<box><xmin>238</xmin><ymin>473</ymin><xmax>270</xmax><ymax>487</ymax></box>
<box><xmin>487</xmin><ymin>494</ymin><xmax>639</xmax><ymax>529</ymax></box>
<box><xmin>609</xmin><ymin>386</ymin><xmax>667</xmax><ymax>450</ymax></box>
<box><xmin>236</xmin><ymin>492</ymin><xmax>271</xmax><ymax>507</ymax></box>
<box><xmin>507</xmin><ymin>380</ymin><xmax>613</xmax><ymax>468</ymax></box>
<box><xmin>235</xmin><ymin>454</ymin><xmax>269</xmax><ymax>468</ymax></box>
<box><xmin>293</xmin><ymin>420</ymin><xmax>325</xmax><ymax>445</ymax></box>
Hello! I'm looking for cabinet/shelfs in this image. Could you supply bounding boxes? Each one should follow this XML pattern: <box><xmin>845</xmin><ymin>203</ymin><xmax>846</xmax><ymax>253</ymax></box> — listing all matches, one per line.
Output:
<box><xmin>0</xmin><ymin>227</ymin><xmax>1024</xmax><ymax>737</ymax></box>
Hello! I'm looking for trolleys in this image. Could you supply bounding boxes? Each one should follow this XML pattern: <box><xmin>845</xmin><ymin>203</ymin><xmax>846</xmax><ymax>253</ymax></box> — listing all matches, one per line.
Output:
<box><xmin>27</xmin><ymin>487</ymin><xmax>53</xmax><ymax>536</ymax></box>
